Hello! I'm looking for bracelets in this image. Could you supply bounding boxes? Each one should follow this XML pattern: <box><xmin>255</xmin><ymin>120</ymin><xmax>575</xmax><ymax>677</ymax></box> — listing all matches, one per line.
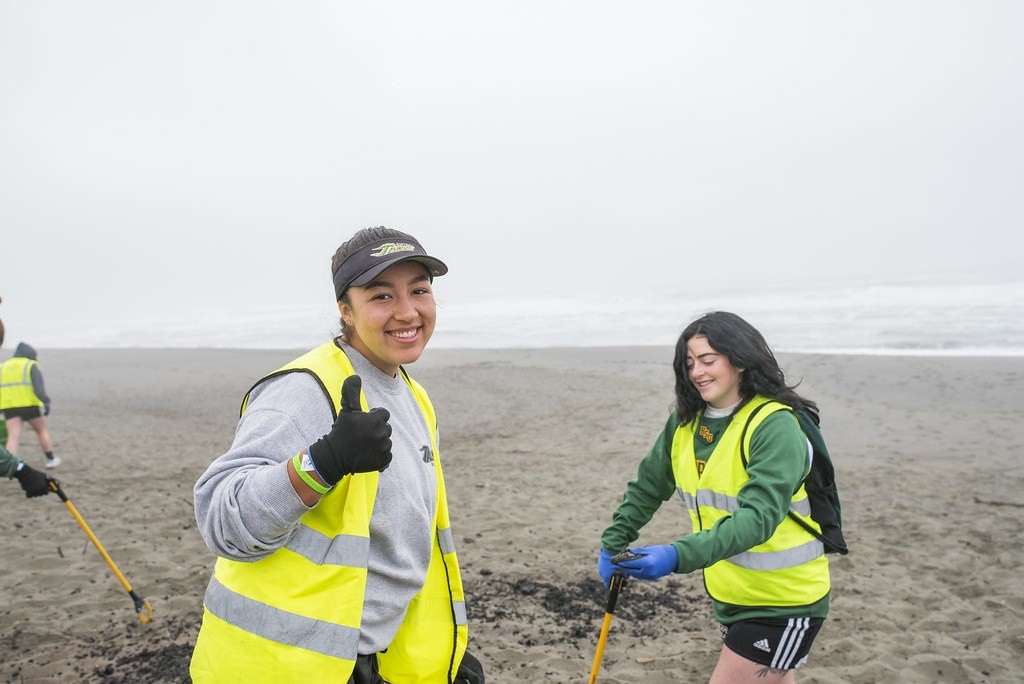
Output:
<box><xmin>293</xmin><ymin>452</ymin><xmax>332</xmax><ymax>494</ymax></box>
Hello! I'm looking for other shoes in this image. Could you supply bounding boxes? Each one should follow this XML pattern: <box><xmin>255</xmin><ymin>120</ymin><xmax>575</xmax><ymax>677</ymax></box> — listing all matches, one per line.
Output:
<box><xmin>46</xmin><ymin>457</ymin><xmax>59</xmax><ymax>467</ymax></box>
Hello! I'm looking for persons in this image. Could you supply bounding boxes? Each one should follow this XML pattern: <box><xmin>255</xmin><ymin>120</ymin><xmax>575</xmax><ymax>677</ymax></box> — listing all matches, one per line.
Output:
<box><xmin>597</xmin><ymin>311</ymin><xmax>832</xmax><ymax>683</ymax></box>
<box><xmin>0</xmin><ymin>318</ymin><xmax>61</xmax><ymax>497</ymax></box>
<box><xmin>189</xmin><ymin>227</ymin><xmax>484</xmax><ymax>683</ymax></box>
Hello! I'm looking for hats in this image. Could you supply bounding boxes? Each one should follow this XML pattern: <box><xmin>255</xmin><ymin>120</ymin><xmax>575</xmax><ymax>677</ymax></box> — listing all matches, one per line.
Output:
<box><xmin>333</xmin><ymin>237</ymin><xmax>448</xmax><ymax>302</ymax></box>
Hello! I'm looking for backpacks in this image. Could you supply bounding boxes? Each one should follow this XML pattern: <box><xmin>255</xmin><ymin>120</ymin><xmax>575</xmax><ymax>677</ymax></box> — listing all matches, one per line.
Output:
<box><xmin>740</xmin><ymin>400</ymin><xmax>848</xmax><ymax>554</ymax></box>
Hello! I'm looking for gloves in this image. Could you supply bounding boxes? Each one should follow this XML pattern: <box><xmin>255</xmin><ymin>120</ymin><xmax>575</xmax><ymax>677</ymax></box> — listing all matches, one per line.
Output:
<box><xmin>14</xmin><ymin>464</ymin><xmax>60</xmax><ymax>498</ymax></box>
<box><xmin>452</xmin><ymin>651</ymin><xmax>485</xmax><ymax>684</ymax></box>
<box><xmin>597</xmin><ymin>548</ymin><xmax>630</xmax><ymax>592</ymax></box>
<box><xmin>307</xmin><ymin>375</ymin><xmax>392</xmax><ymax>487</ymax></box>
<box><xmin>617</xmin><ymin>543</ymin><xmax>678</xmax><ymax>580</ymax></box>
